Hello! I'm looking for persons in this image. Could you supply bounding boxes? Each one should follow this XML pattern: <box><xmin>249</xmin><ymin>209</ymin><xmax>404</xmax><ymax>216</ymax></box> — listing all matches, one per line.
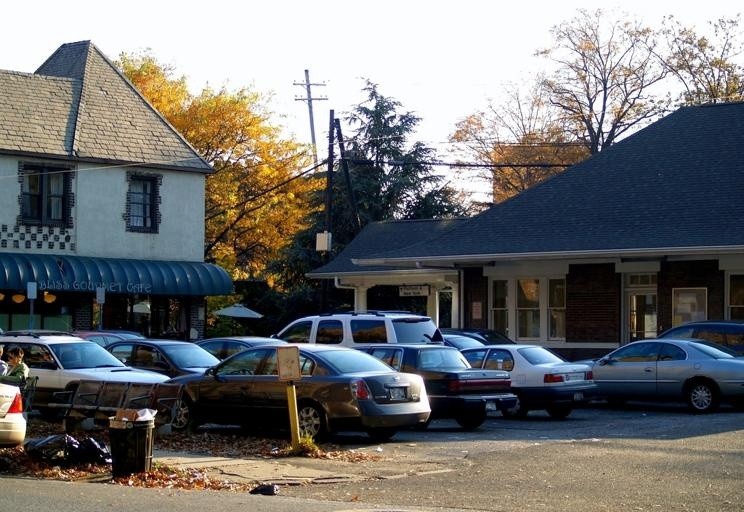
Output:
<box><xmin>8</xmin><ymin>345</ymin><xmax>30</xmax><ymax>394</ymax></box>
<box><xmin>38</xmin><ymin>347</ymin><xmax>54</xmax><ymax>368</ymax></box>
<box><xmin>0</xmin><ymin>344</ymin><xmax>9</xmax><ymax>376</ymax></box>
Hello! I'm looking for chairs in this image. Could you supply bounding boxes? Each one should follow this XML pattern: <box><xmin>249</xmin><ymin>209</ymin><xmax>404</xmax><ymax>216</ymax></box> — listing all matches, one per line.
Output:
<box><xmin>48</xmin><ymin>378</ymin><xmax>186</xmax><ymax>442</ymax></box>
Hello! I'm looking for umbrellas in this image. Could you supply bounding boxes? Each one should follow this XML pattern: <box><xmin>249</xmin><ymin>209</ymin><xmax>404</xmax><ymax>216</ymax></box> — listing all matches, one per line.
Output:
<box><xmin>213</xmin><ymin>301</ymin><xmax>264</xmax><ymax>323</ymax></box>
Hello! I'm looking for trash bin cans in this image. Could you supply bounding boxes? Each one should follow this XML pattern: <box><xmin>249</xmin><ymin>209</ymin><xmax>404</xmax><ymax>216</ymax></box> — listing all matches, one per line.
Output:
<box><xmin>108</xmin><ymin>416</ymin><xmax>155</xmax><ymax>477</ymax></box>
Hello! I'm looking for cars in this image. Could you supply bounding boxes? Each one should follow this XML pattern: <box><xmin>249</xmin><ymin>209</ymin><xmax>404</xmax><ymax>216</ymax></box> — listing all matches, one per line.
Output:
<box><xmin>571</xmin><ymin>339</ymin><xmax>744</xmax><ymax>413</ymax></box>
<box><xmin>658</xmin><ymin>321</ymin><xmax>744</xmax><ymax>355</ymax></box>
